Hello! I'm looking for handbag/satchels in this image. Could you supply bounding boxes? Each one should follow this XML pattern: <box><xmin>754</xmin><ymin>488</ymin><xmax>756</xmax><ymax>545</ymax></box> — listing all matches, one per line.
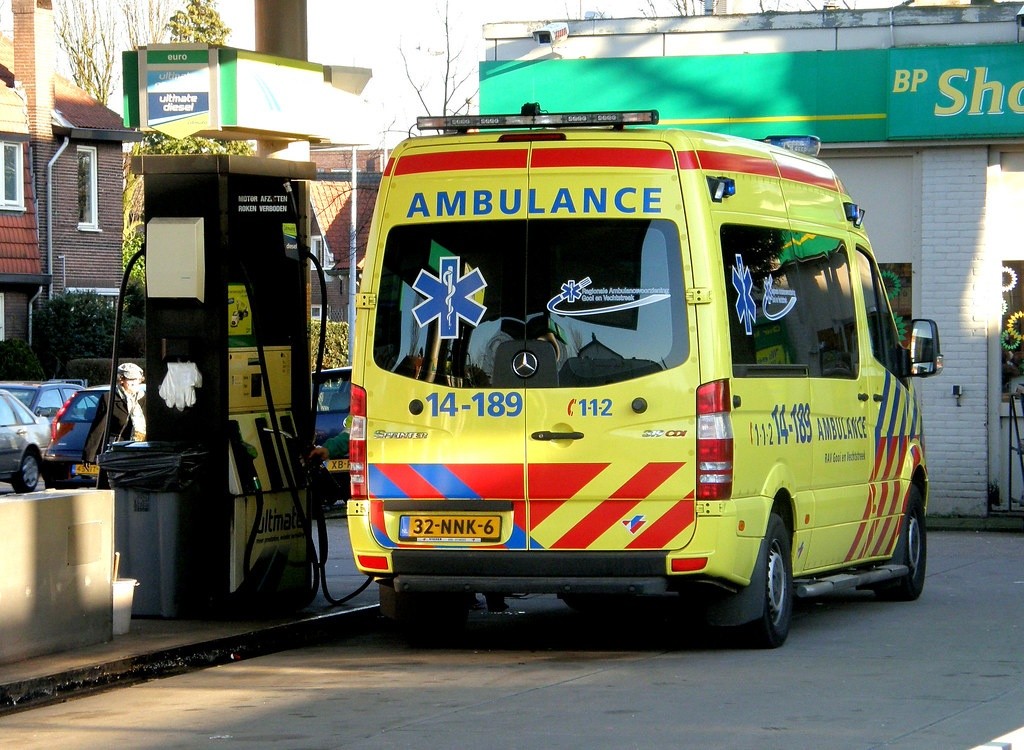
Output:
<box><xmin>107</xmin><ymin>433</ymin><xmax>121</xmax><ymax>451</ymax></box>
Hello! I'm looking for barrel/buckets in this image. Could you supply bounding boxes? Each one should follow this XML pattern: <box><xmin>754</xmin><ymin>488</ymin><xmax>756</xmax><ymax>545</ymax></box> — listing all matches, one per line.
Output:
<box><xmin>112</xmin><ymin>578</ymin><xmax>140</xmax><ymax>636</ymax></box>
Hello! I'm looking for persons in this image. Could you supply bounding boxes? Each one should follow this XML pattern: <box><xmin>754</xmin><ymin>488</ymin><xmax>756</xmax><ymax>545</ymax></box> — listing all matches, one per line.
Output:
<box><xmin>309</xmin><ymin>411</ymin><xmax>352</xmax><ymax>460</ymax></box>
<box><xmin>81</xmin><ymin>362</ymin><xmax>147</xmax><ymax>471</ymax></box>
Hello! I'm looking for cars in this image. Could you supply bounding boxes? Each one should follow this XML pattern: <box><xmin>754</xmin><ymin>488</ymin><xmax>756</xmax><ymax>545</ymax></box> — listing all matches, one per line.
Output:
<box><xmin>42</xmin><ymin>384</ymin><xmax>147</xmax><ymax>490</ymax></box>
<box><xmin>0</xmin><ymin>378</ymin><xmax>88</xmax><ymax>422</ymax></box>
<box><xmin>310</xmin><ymin>367</ymin><xmax>352</xmax><ymax>515</ymax></box>
<box><xmin>0</xmin><ymin>389</ymin><xmax>51</xmax><ymax>493</ymax></box>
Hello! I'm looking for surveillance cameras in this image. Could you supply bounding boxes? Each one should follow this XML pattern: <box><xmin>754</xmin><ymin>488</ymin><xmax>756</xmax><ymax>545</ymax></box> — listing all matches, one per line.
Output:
<box><xmin>532</xmin><ymin>22</ymin><xmax>570</xmax><ymax>46</ymax></box>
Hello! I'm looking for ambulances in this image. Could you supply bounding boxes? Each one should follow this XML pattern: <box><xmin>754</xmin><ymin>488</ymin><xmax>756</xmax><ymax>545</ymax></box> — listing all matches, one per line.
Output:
<box><xmin>346</xmin><ymin>105</ymin><xmax>943</xmax><ymax>652</ymax></box>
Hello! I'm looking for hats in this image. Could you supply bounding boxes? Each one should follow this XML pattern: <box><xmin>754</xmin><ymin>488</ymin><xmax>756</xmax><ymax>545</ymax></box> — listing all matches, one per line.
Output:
<box><xmin>117</xmin><ymin>363</ymin><xmax>143</xmax><ymax>380</ymax></box>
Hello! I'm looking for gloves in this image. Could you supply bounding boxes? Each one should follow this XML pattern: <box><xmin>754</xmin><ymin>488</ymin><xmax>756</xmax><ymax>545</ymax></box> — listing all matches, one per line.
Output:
<box><xmin>159</xmin><ymin>362</ymin><xmax>202</xmax><ymax>411</ymax></box>
